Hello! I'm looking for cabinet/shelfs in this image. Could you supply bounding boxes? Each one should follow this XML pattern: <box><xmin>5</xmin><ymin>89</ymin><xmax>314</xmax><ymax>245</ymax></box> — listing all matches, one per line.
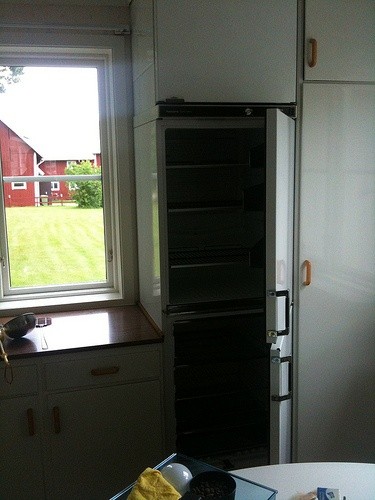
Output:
<box><xmin>0</xmin><ymin>304</ymin><xmax>165</xmax><ymax>500</ymax></box>
<box><xmin>129</xmin><ymin>0</ymin><xmax>375</xmax><ymax>462</ymax></box>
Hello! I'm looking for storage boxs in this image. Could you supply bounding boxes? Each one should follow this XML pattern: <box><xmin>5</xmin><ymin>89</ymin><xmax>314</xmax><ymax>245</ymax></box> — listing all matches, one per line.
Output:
<box><xmin>107</xmin><ymin>452</ymin><xmax>276</xmax><ymax>500</ymax></box>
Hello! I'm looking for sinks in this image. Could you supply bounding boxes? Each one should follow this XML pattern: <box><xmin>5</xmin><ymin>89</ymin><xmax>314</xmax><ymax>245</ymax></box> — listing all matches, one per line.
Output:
<box><xmin>228</xmin><ymin>463</ymin><xmax>375</xmax><ymax>500</ymax></box>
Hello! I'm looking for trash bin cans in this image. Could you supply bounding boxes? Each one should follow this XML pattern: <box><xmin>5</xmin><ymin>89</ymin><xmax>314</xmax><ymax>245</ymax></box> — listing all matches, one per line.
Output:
<box><xmin>41</xmin><ymin>195</ymin><xmax>48</xmax><ymax>206</ymax></box>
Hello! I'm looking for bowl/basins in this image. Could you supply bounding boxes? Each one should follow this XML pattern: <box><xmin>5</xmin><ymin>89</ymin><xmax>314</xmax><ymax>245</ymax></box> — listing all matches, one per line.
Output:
<box><xmin>2</xmin><ymin>313</ymin><xmax>36</xmax><ymax>339</ymax></box>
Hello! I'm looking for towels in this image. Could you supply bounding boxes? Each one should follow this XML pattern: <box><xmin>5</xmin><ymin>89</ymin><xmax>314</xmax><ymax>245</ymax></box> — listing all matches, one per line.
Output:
<box><xmin>128</xmin><ymin>467</ymin><xmax>181</xmax><ymax>500</ymax></box>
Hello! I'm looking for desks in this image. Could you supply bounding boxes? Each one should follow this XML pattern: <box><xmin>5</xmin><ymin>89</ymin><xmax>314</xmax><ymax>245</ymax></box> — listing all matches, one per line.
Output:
<box><xmin>228</xmin><ymin>461</ymin><xmax>375</xmax><ymax>500</ymax></box>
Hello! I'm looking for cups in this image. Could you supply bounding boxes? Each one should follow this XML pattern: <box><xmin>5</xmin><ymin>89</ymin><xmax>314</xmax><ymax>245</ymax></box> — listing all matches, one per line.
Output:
<box><xmin>188</xmin><ymin>470</ymin><xmax>237</xmax><ymax>500</ymax></box>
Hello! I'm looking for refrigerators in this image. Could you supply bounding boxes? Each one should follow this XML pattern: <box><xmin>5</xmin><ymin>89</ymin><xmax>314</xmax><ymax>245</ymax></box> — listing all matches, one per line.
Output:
<box><xmin>129</xmin><ymin>105</ymin><xmax>296</xmax><ymax>465</ymax></box>
